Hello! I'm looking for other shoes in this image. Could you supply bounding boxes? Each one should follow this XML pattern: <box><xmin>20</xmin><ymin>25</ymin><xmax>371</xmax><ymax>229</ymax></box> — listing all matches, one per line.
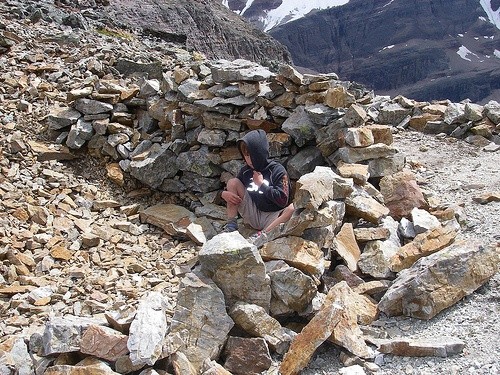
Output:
<box><xmin>223</xmin><ymin>222</ymin><xmax>239</xmax><ymax>232</ymax></box>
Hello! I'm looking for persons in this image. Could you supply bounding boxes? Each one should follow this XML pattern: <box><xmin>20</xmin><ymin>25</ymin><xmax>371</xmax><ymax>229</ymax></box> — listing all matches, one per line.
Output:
<box><xmin>215</xmin><ymin>129</ymin><xmax>295</xmax><ymax>242</ymax></box>
